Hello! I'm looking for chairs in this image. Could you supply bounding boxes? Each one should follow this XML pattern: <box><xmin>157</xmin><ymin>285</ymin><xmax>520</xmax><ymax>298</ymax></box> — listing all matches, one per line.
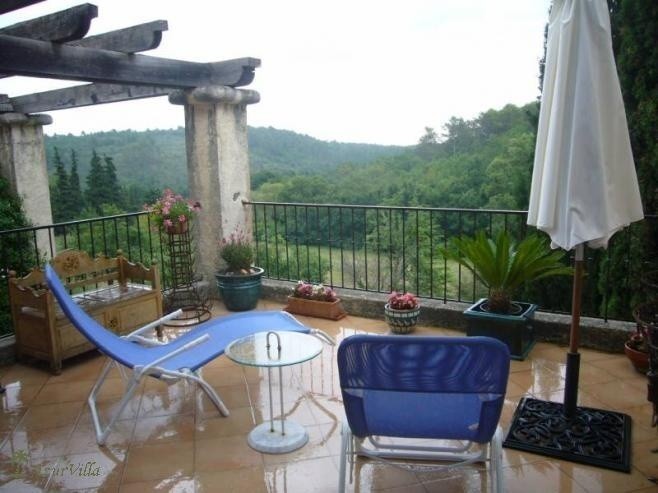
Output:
<box><xmin>338</xmin><ymin>335</ymin><xmax>510</xmax><ymax>493</ymax></box>
<box><xmin>44</xmin><ymin>262</ymin><xmax>336</xmax><ymax>446</ymax></box>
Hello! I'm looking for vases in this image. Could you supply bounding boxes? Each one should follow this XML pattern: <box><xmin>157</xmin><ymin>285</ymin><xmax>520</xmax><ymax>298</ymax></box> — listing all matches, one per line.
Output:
<box><xmin>282</xmin><ymin>294</ymin><xmax>348</xmax><ymax>321</ymax></box>
<box><xmin>623</xmin><ymin>341</ymin><xmax>650</xmax><ymax>373</ymax></box>
<box><xmin>166</xmin><ymin>221</ymin><xmax>188</xmax><ymax>233</ymax></box>
<box><xmin>385</xmin><ymin>303</ymin><xmax>420</xmax><ymax>334</ymax></box>
<box><xmin>214</xmin><ymin>267</ymin><xmax>265</xmax><ymax>310</ymax></box>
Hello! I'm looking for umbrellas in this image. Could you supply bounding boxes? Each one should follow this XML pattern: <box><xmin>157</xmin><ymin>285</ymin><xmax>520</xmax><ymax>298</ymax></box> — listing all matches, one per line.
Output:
<box><xmin>524</xmin><ymin>0</ymin><xmax>645</xmax><ymax>419</ymax></box>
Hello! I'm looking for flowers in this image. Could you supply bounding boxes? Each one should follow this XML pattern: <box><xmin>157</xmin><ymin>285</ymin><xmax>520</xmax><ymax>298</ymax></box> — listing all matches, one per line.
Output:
<box><xmin>387</xmin><ymin>291</ymin><xmax>417</xmax><ymax>308</ymax></box>
<box><xmin>627</xmin><ymin>326</ymin><xmax>651</xmax><ymax>351</ymax></box>
<box><xmin>142</xmin><ymin>189</ymin><xmax>203</xmax><ymax>234</ymax></box>
<box><xmin>292</xmin><ymin>280</ymin><xmax>337</xmax><ymax>302</ymax></box>
<box><xmin>212</xmin><ymin>220</ymin><xmax>258</xmax><ymax>272</ymax></box>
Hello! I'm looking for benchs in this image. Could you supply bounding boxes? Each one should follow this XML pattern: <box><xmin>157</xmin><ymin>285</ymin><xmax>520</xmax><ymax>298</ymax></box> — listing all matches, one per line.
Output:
<box><xmin>8</xmin><ymin>248</ymin><xmax>164</xmax><ymax>376</ymax></box>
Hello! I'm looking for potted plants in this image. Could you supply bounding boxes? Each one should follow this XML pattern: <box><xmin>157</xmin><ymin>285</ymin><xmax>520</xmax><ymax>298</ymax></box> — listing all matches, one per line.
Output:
<box><xmin>436</xmin><ymin>223</ymin><xmax>574</xmax><ymax>361</ymax></box>
